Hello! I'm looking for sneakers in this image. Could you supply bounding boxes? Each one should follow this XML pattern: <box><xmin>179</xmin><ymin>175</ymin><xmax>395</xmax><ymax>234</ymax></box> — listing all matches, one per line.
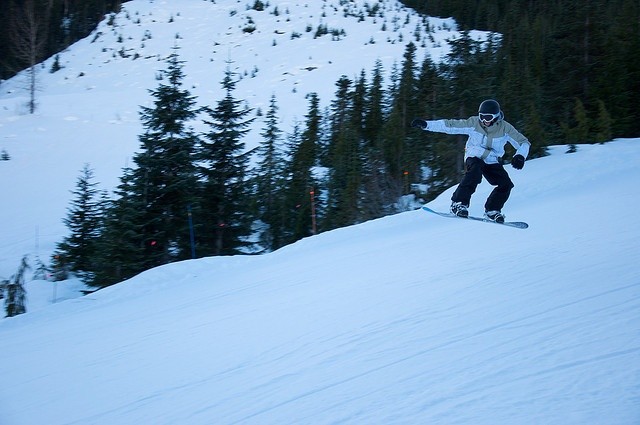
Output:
<box><xmin>450</xmin><ymin>200</ymin><xmax>468</xmax><ymax>217</ymax></box>
<box><xmin>483</xmin><ymin>209</ymin><xmax>504</xmax><ymax>223</ymax></box>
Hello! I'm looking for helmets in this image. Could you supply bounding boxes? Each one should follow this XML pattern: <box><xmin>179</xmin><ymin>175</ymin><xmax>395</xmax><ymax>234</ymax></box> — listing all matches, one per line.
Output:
<box><xmin>478</xmin><ymin>98</ymin><xmax>500</xmax><ymax>114</ymax></box>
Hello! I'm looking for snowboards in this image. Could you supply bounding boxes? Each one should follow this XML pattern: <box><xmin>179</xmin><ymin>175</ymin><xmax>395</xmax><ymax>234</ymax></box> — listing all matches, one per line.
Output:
<box><xmin>423</xmin><ymin>207</ymin><xmax>529</xmax><ymax>230</ymax></box>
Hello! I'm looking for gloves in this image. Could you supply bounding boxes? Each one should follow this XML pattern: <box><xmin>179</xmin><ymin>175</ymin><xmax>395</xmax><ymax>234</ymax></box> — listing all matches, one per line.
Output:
<box><xmin>411</xmin><ymin>117</ymin><xmax>427</xmax><ymax>127</ymax></box>
<box><xmin>510</xmin><ymin>153</ymin><xmax>525</xmax><ymax>169</ymax></box>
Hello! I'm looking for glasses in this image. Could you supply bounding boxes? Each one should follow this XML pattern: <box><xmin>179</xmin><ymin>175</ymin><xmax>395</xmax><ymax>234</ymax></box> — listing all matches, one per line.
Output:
<box><xmin>478</xmin><ymin>111</ymin><xmax>500</xmax><ymax>122</ymax></box>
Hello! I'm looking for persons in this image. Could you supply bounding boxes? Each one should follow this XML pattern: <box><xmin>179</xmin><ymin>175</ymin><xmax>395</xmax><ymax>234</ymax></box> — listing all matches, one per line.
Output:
<box><xmin>411</xmin><ymin>99</ymin><xmax>531</xmax><ymax>224</ymax></box>
